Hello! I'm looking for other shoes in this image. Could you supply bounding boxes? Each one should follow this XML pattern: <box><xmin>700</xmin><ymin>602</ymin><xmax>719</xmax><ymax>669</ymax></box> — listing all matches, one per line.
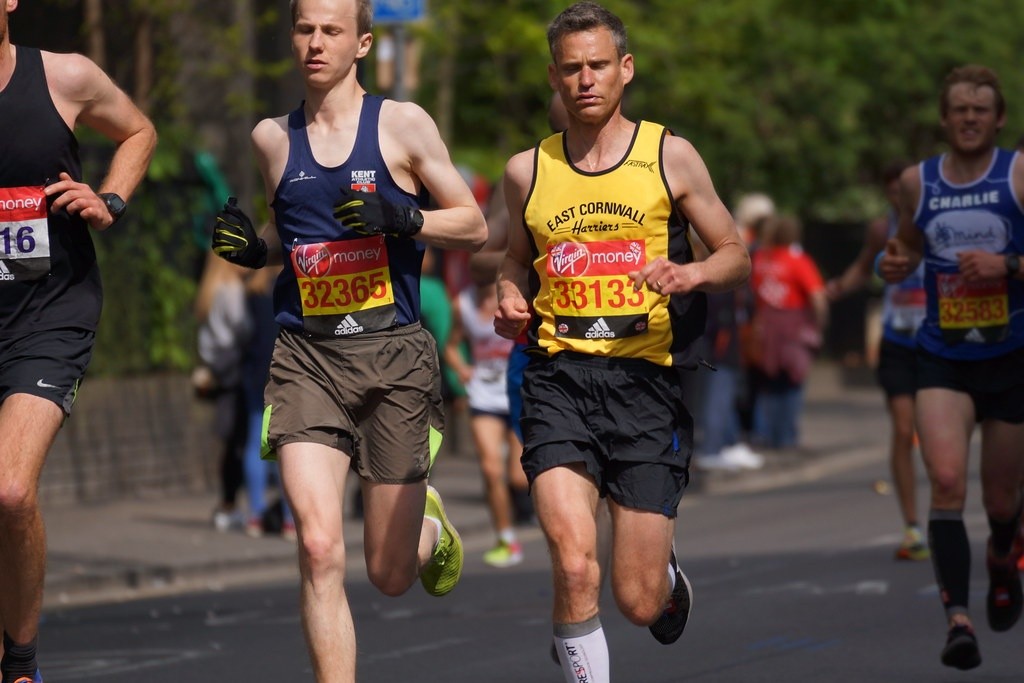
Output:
<box><xmin>212</xmin><ymin>509</ymin><xmax>301</xmax><ymax>542</ymax></box>
<box><xmin>695</xmin><ymin>441</ymin><xmax>764</xmax><ymax>471</ymax></box>
<box><xmin>897</xmin><ymin>528</ymin><xmax>933</xmax><ymax>561</ymax></box>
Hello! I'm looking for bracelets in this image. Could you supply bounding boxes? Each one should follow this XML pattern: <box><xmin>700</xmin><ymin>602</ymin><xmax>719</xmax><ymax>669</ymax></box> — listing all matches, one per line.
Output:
<box><xmin>875</xmin><ymin>253</ymin><xmax>886</xmax><ymax>274</ymax></box>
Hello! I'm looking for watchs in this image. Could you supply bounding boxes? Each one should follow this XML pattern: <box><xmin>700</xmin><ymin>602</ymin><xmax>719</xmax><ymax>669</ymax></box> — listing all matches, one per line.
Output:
<box><xmin>405</xmin><ymin>206</ymin><xmax>424</xmax><ymax>235</ymax></box>
<box><xmin>96</xmin><ymin>192</ymin><xmax>127</xmax><ymax>224</ymax></box>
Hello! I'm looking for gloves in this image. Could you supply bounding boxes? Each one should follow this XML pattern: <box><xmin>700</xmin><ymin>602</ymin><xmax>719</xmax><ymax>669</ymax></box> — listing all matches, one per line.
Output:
<box><xmin>211</xmin><ymin>203</ymin><xmax>268</xmax><ymax>269</ymax></box>
<box><xmin>332</xmin><ymin>187</ymin><xmax>424</xmax><ymax>237</ymax></box>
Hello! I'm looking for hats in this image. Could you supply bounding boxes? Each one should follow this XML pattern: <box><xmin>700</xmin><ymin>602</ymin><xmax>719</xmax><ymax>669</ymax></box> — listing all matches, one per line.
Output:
<box><xmin>735</xmin><ymin>192</ymin><xmax>776</xmax><ymax>226</ymax></box>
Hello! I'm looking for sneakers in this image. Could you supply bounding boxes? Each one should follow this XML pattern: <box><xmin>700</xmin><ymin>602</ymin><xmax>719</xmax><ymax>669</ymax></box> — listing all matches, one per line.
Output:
<box><xmin>420</xmin><ymin>485</ymin><xmax>463</xmax><ymax>597</ymax></box>
<box><xmin>482</xmin><ymin>538</ymin><xmax>522</xmax><ymax>568</ymax></box>
<box><xmin>648</xmin><ymin>535</ymin><xmax>693</xmax><ymax>645</ymax></box>
<box><xmin>986</xmin><ymin>535</ymin><xmax>1024</xmax><ymax>632</ymax></box>
<box><xmin>941</xmin><ymin>613</ymin><xmax>982</xmax><ymax>670</ymax></box>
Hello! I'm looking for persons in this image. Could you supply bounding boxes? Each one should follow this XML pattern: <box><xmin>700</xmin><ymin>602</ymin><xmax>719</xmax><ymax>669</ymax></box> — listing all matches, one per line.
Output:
<box><xmin>211</xmin><ymin>0</ymin><xmax>488</xmax><ymax>683</ymax></box>
<box><xmin>194</xmin><ymin>258</ymin><xmax>298</xmax><ymax>541</ymax></box>
<box><xmin>494</xmin><ymin>0</ymin><xmax>752</xmax><ymax>683</ymax></box>
<box><xmin>681</xmin><ymin>194</ymin><xmax>830</xmax><ymax>473</ymax></box>
<box><xmin>876</xmin><ymin>66</ymin><xmax>1024</xmax><ymax>671</ymax></box>
<box><xmin>418</xmin><ymin>174</ymin><xmax>537</xmax><ymax>565</ymax></box>
<box><xmin>0</xmin><ymin>0</ymin><xmax>157</xmax><ymax>683</ymax></box>
<box><xmin>824</xmin><ymin>162</ymin><xmax>927</xmax><ymax>562</ymax></box>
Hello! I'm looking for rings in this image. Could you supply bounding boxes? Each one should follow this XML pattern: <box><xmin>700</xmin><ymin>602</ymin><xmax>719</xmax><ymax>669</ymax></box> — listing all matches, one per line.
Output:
<box><xmin>656</xmin><ymin>280</ymin><xmax>662</xmax><ymax>288</ymax></box>
